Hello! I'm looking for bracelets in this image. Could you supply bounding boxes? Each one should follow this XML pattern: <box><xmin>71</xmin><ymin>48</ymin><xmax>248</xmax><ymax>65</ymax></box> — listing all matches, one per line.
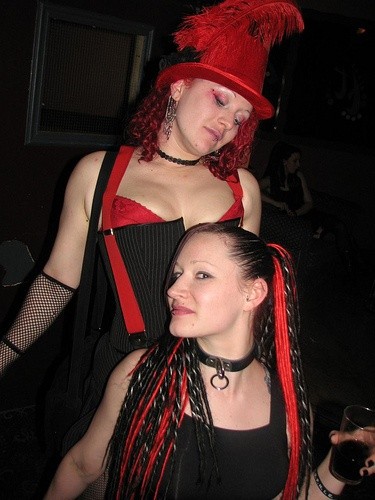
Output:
<box><xmin>292</xmin><ymin>210</ymin><xmax>298</xmax><ymax>217</ymax></box>
<box><xmin>312</xmin><ymin>468</ymin><xmax>341</xmax><ymax>499</ymax></box>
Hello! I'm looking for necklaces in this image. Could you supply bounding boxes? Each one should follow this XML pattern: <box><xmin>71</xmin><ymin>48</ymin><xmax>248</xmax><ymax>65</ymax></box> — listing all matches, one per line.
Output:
<box><xmin>156</xmin><ymin>148</ymin><xmax>201</xmax><ymax>165</ymax></box>
<box><xmin>197</xmin><ymin>346</ymin><xmax>258</xmax><ymax>390</ymax></box>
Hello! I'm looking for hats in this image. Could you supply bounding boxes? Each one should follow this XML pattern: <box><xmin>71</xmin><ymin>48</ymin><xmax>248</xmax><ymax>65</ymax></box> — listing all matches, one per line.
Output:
<box><xmin>156</xmin><ymin>34</ymin><xmax>275</xmax><ymax>120</ymax></box>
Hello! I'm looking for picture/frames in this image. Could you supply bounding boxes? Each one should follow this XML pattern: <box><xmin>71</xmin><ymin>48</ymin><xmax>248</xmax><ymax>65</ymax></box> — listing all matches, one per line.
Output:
<box><xmin>23</xmin><ymin>4</ymin><xmax>154</xmax><ymax>151</ymax></box>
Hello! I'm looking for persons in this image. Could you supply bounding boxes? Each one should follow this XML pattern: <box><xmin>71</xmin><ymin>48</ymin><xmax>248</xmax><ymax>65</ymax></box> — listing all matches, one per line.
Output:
<box><xmin>258</xmin><ymin>142</ymin><xmax>375</xmax><ymax>295</ymax></box>
<box><xmin>45</xmin><ymin>222</ymin><xmax>375</xmax><ymax>500</ymax></box>
<box><xmin>0</xmin><ymin>0</ymin><xmax>306</xmax><ymax>500</ymax></box>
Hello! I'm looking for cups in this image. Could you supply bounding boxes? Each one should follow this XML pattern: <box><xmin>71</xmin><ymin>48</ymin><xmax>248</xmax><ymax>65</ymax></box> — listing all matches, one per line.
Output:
<box><xmin>328</xmin><ymin>405</ymin><xmax>375</xmax><ymax>484</ymax></box>
